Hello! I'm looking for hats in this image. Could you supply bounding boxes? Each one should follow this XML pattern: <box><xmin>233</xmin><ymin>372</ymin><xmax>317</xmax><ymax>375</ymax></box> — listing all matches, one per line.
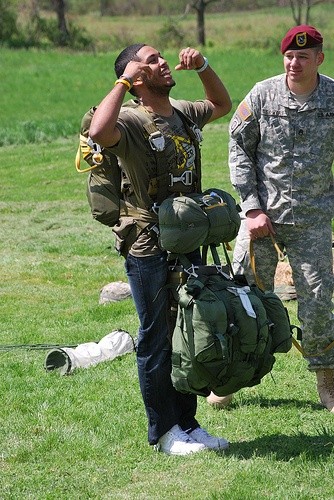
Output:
<box><xmin>281</xmin><ymin>25</ymin><xmax>324</xmax><ymax>53</ymax></box>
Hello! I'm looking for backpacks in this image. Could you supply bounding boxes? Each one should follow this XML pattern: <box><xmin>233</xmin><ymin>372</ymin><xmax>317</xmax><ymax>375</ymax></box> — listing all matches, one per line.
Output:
<box><xmin>75</xmin><ymin>97</ymin><xmax>203</xmax><ymax>228</ymax></box>
<box><xmin>170</xmin><ymin>268</ymin><xmax>293</xmax><ymax>397</ymax></box>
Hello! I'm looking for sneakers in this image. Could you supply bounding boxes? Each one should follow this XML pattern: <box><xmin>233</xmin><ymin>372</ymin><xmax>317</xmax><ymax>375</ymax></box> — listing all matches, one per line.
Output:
<box><xmin>154</xmin><ymin>424</ymin><xmax>205</xmax><ymax>456</ymax></box>
<box><xmin>189</xmin><ymin>428</ymin><xmax>228</xmax><ymax>450</ymax></box>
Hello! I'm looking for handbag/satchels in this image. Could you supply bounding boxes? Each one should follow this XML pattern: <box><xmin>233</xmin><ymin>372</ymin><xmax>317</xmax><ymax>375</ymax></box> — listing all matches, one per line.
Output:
<box><xmin>159</xmin><ymin>189</ymin><xmax>242</xmax><ymax>254</ymax></box>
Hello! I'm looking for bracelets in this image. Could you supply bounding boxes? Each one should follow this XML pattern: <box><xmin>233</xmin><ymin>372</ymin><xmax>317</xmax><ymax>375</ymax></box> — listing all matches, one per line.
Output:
<box><xmin>114</xmin><ymin>79</ymin><xmax>130</xmax><ymax>91</ymax></box>
<box><xmin>194</xmin><ymin>56</ymin><xmax>209</xmax><ymax>73</ymax></box>
<box><xmin>119</xmin><ymin>74</ymin><xmax>133</xmax><ymax>88</ymax></box>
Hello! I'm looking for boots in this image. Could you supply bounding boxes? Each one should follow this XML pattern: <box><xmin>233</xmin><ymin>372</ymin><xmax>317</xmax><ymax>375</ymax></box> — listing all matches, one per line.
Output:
<box><xmin>207</xmin><ymin>391</ymin><xmax>231</xmax><ymax>409</ymax></box>
<box><xmin>316</xmin><ymin>369</ymin><xmax>334</xmax><ymax>413</ymax></box>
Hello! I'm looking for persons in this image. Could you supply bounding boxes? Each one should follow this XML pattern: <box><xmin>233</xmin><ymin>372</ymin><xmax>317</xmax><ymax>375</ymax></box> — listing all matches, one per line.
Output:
<box><xmin>206</xmin><ymin>23</ymin><xmax>334</xmax><ymax>414</ymax></box>
<box><xmin>88</xmin><ymin>42</ymin><xmax>233</xmax><ymax>459</ymax></box>
